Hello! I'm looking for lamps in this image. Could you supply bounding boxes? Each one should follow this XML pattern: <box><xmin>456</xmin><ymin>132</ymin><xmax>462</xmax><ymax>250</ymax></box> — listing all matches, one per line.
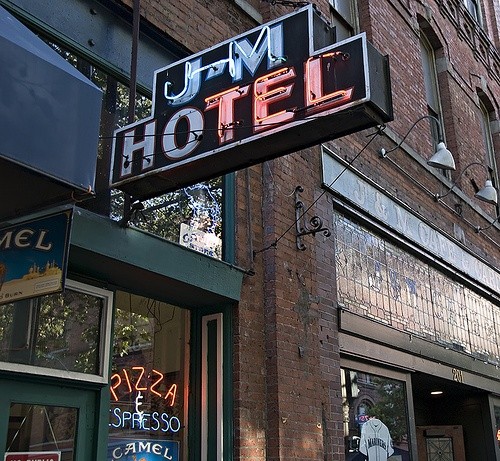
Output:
<box><xmin>435</xmin><ymin>162</ymin><xmax>498</xmax><ymax>205</ymax></box>
<box><xmin>382</xmin><ymin>115</ymin><xmax>457</xmax><ymax>171</ymax></box>
